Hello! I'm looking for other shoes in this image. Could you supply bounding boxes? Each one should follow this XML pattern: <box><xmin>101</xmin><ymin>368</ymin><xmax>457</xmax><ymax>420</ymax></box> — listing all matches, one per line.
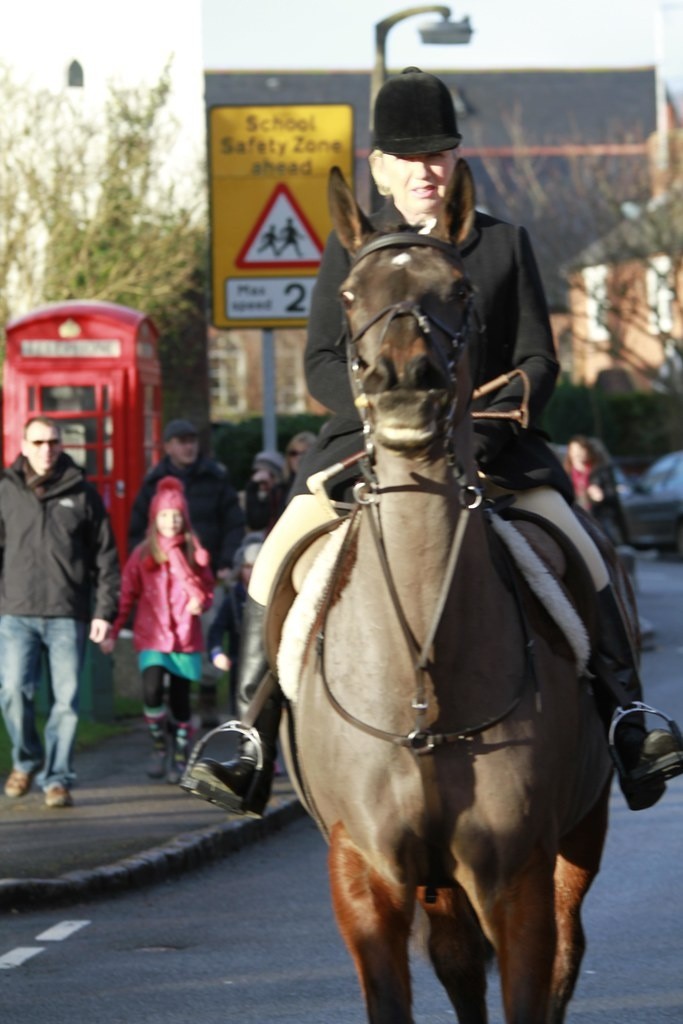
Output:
<box><xmin>44</xmin><ymin>779</ymin><xmax>73</xmax><ymax>808</ymax></box>
<box><xmin>168</xmin><ymin>754</ymin><xmax>190</xmax><ymax>785</ymax></box>
<box><xmin>147</xmin><ymin>750</ymin><xmax>166</xmax><ymax>776</ymax></box>
<box><xmin>3</xmin><ymin>754</ymin><xmax>44</xmax><ymax>798</ymax></box>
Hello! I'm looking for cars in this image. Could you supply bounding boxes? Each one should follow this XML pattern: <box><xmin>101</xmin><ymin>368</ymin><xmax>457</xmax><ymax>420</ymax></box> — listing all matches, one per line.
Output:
<box><xmin>616</xmin><ymin>446</ymin><xmax>683</xmax><ymax>559</ymax></box>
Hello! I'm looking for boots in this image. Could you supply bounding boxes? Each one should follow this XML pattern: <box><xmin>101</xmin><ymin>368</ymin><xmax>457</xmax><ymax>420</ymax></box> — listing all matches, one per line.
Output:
<box><xmin>584</xmin><ymin>583</ymin><xmax>674</xmax><ymax>811</ymax></box>
<box><xmin>189</xmin><ymin>590</ymin><xmax>287</xmax><ymax>819</ymax></box>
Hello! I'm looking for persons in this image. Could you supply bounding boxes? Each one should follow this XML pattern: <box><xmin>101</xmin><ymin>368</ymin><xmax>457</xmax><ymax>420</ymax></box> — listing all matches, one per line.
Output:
<box><xmin>561</xmin><ymin>435</ymin><xmax>630</xmax><ymax>546</ymax></box>
<box><xmin>99</xmin><ymin>418</ymin><xmax>317</xmax><ymax>784</ymax></box>
<box><xmin>188</xmin><ymin>65</ymin><xmax>683</xmax><ymax>821</ymax></box>
<box><xmin>0</xmin><ymin>416</ymin><xmax>122</xmax><ymax>808</ymax></box>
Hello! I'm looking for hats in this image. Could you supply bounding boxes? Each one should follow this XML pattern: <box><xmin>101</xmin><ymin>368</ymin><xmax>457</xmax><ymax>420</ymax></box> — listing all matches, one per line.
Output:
<box><xmin>164</xmin><ymin>419</ymin><xmax>200</xmax><ymax>439</ymax></box>
<box><xmin>146</xmin><ymin>477</ymin><xmax>208</xmax><ymax>568</ymax></box>
<box><xmin>242</xmin><ymin>543</ymin><xmax>261</xmax><ymax>563</ymax></box>
<box><xmin>255</xmin><ymin>451</ymin><xmax>285</xmax><ymax>482</ymax></box>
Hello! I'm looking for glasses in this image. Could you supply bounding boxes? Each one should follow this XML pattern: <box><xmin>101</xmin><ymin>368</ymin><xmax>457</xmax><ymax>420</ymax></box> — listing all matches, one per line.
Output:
<box><xmin>27</xmin><ymin>439</ymin><xmax>63</xmax><ymax>445</ymax></box>
<box><xmin>287</xmin><ymin>449</ymin><xmax>307</xmax><ymax>459</ymax></box>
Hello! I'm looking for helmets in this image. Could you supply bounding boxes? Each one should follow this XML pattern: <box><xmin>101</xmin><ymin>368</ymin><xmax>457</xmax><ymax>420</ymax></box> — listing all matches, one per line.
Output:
<box><xmin>372</xmin><ymin>68</ymin><xmax>462</xmax><ymax>158</ymax></box>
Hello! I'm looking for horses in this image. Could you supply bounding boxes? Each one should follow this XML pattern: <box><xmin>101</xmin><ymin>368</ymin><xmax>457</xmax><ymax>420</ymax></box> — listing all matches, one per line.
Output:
<box><xmin>237</xmin><ymin>156</ymin><xmax>642</xmax><ymax>1024</ymax></box>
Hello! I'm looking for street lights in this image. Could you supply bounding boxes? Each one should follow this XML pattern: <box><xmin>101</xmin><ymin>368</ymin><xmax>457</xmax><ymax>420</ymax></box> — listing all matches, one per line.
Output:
<box><xmin>371</xmin><ymin>6</ymin><xmax>474</xmax><ymax>162</ymax></box>
